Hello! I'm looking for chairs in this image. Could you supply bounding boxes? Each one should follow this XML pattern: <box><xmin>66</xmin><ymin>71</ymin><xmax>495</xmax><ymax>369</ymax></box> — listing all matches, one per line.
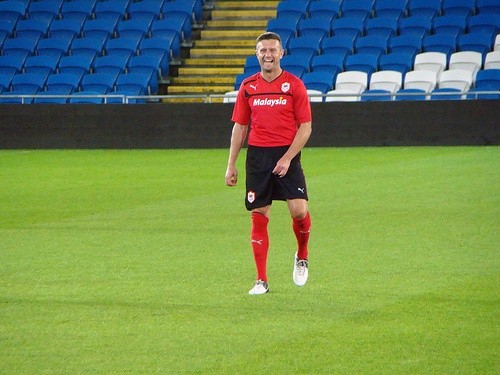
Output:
<box><xmin>0</xmin><ymin>0</ymin><xmax>500</xmax><ymax>105</ymax></box>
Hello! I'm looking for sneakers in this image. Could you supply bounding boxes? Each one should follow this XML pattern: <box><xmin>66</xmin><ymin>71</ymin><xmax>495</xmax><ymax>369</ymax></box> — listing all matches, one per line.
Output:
<box><xmin>293</xmin><ymin>251</ymin><xmax>309</xmax><ymax>287</ymax></box>
<box><xmin>249</xmin><ymin>278</ymin><xmax>269</xmax><ymax>295</ymax></box>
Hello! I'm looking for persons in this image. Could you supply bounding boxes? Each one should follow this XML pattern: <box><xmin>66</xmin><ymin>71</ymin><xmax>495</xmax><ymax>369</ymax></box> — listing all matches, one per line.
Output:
<box><xmin>224</xmin><ymin>31</ymin><xmax>311</xmax><ymax>295</ymax></box>
